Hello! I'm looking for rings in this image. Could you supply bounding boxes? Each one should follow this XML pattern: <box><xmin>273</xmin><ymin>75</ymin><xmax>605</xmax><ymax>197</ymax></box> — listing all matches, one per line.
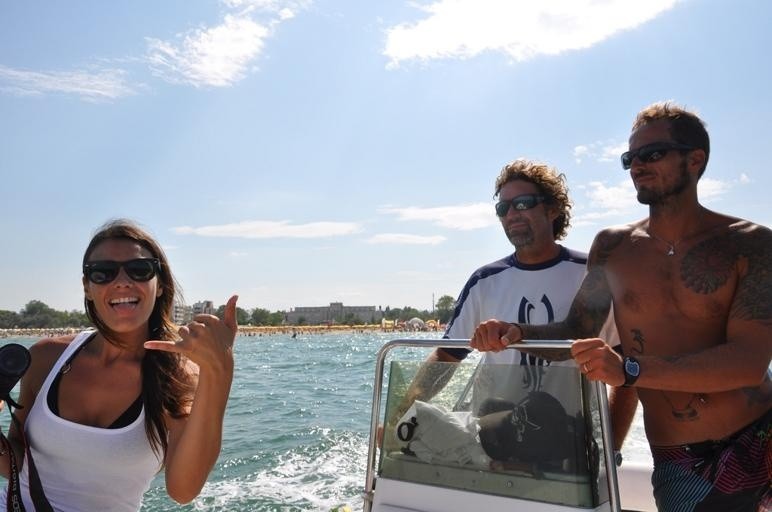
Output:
<box><xmin>583</xmin><ymin>363</ymin><xmax>589</xmax><ymax>372</ymax></box>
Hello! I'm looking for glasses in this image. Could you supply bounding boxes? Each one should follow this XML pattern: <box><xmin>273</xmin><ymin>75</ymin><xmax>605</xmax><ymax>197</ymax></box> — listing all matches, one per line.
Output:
<box><xmin>620</xmin><ymin>141</ymin><xmax>701</xmax><ymax>171</ymax></box>
<box><xmin>82</xmin><ymin>257</ymin><xmax>164</xmax><ymax>285</ymax></box>
<box><xmin>494</xmin><ymin>193</ymin><xmax>552</xmax><ymax>217</ymax></box>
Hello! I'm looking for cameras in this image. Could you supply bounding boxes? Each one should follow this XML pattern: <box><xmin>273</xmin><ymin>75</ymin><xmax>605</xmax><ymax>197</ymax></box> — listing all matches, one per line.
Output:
<box><xmin>0</xmin><ymin>343</ymin><xmax>31</xmax><ymax>399</ymax></box>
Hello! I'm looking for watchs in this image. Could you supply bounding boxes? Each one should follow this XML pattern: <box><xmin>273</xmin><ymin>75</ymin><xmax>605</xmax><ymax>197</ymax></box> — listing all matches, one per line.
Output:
<box><xmin>614</xmin><ymin>451</ymin><xmax>623</xmax><ymax>468</ymax></box>
<box><xmin>621</xmin><ymin>354</ymin><xmax>641</xmax><ymax>389</ymax></box>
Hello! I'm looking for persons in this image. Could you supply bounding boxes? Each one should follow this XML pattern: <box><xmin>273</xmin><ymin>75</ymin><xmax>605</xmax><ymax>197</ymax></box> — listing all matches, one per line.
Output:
<box><xmin>470</xmin><ymin>99</ymin><xmax>772</xmax><ymax>512</ymax></box>
<box><xmin>373</xmin><ymin>157</ymin><xmax>638</xmax><ymax>472</ymax></box>
<box><xmin>0</xmin><ymin>217</ymin><xmax>240</xmax><ymax>512</ymax></box>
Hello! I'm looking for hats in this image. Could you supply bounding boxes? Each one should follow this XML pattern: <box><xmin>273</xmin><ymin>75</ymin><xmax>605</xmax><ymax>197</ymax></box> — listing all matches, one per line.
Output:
<box><xmin>479</xmin><ymin>392</ymin><xmax>572</xmax><ymax>462</ymax></box>
